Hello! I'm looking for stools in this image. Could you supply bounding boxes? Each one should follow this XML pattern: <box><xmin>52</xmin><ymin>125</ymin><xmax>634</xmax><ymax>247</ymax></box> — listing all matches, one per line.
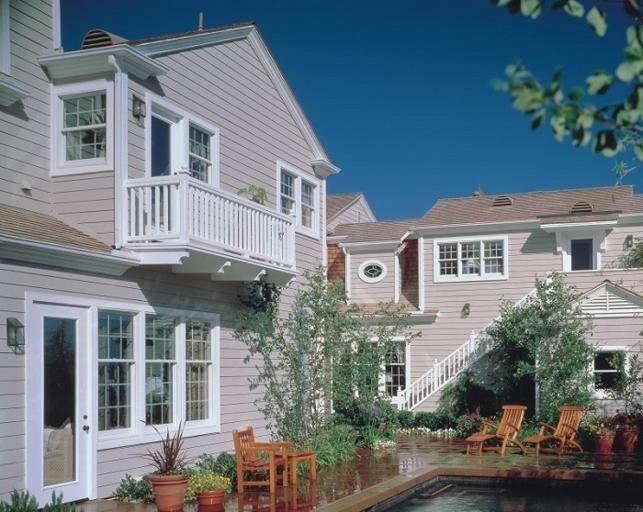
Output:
<box><xmin>272</xmin><ymin>450</ymin><xmax>317</xmax><ymax>484</ymax></box>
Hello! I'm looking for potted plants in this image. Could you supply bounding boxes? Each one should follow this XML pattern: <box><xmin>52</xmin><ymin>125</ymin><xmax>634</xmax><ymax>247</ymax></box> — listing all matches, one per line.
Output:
<box><xmin>588</xmin><ymin>350</ymin><xmax>643</xmax><ymax>454</ymax></box>
<box><xmin>128</xmin><ymin>419</ymin><xmax>231</xmax><ymax>512</ymax></box>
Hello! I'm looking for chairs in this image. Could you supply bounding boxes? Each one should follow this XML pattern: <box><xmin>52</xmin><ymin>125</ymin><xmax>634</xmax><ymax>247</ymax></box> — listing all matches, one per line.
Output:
<box><xmin>465</xmin><ymin>405</ymin><xmax>584</xmax><ymax>457</ymax></box>
<box><xmin>232</xmin><ymin>427</ymin><xmax>290</xmax><ymax>494</ymax></box>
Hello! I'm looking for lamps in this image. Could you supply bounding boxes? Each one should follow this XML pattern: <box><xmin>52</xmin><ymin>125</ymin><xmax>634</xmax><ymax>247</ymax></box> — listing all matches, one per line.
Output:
<box><xmin>133</xmin><ymin>95</ymin><xmax>146</xmax><ymax>117</ymax></box>
<box><xmin>8</xmin><ymin>318</ymin><xmax>24</xmax><ymax>347</ymax></box>
